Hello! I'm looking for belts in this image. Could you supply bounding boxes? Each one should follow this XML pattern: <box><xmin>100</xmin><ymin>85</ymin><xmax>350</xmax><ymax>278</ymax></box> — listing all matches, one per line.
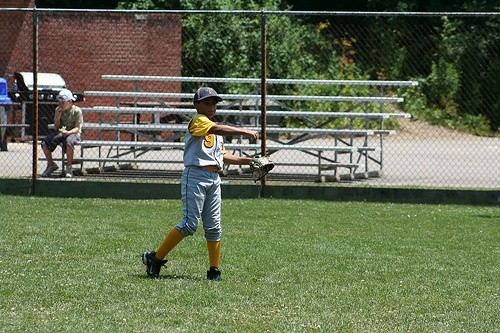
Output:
<box><xmin>202</xmin><ymin>165</ymin><xmax>221</xmax><ymax>172</ymax></box>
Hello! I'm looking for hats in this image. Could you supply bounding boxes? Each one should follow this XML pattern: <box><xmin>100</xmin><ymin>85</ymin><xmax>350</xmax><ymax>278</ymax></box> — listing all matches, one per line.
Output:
<box><xmin>194</xmin><ymin>87</ymin><xmax>222</xmax><ymax>104</ymax></box>
<box><xmin>57</xmin><ymin>88</ymin><xmax>76</xmax><ymax>101</ymax></box>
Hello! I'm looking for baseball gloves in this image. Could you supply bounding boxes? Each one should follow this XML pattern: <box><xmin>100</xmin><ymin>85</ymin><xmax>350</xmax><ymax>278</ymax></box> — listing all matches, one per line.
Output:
<box><xmin>249</xmin><ymin>155</ymin><xmax>275</xmax><ymax>183</ymax></box>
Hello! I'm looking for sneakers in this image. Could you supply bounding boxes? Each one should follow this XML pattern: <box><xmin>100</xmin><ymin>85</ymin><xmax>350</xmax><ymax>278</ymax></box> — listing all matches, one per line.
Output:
<box><xmin>142</xmin><ymin>251</ymin><xmax>168</xmax><ymax>279</ymax></box>
<box><xmin>207</xmin><ymin>269</ymin><xmax>222</xmax><ymax>281</ymax></box>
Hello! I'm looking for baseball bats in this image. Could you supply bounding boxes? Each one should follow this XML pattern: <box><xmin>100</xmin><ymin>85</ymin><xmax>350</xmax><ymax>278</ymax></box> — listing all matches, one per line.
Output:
<box><xmin>61</xmin><ymin>126</ymin><xmax>66</xmax><ymax>175</ymax></box>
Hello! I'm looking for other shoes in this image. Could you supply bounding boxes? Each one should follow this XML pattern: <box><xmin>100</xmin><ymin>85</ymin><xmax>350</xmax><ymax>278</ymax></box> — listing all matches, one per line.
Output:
<box><xmin>42</xmin><ymin>164</ymin><xmax>59</xmax><ymax>177</ymax></box>
<box><xmin>65</xmin><ymin>166</ymin><xmax>73</xmax><ymax>177</ymax></box>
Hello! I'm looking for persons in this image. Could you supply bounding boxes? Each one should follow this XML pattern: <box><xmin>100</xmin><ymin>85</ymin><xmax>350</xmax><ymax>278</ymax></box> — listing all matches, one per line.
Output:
<box><xmin>142</xmin><ymin>87</ymin><xmax>274</xmax><ymax>280</ymax></box>
<box><xmin>42</xmin><ymin>90</ymin><xmax>83</xmax><ymax>177</ymax></box>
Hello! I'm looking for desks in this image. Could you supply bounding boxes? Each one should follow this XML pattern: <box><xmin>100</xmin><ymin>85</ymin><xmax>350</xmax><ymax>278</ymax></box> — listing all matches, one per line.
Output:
<box><xmin>10</xmin><ymin>101</ymin><xmax>59</xmax><ymax>143</ymax></box>
<box><xmin>119</xmin><ymin>101</ymin><xmax>281</xmax><ymax>151</ymax></box>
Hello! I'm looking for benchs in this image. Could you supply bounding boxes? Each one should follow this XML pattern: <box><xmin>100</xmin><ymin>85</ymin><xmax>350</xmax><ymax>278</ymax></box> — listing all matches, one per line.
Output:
<box><xmin>39</xmin><ymin>74</ymin><xmax>419</xmax><ymax>183</ymax></box>
<box><xmin>0</xmin><ymin>124</ymin><xmax>30</xmax><ymax>152</ymax></box>
